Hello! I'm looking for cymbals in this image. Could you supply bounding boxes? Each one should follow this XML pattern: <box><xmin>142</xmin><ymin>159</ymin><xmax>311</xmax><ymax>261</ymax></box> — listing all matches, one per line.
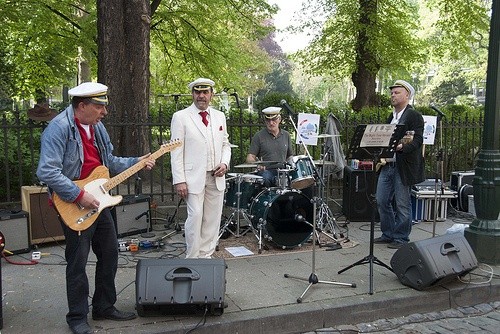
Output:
<box><xmin>307</xmin><ymin>134</ymin><xmax>344</xmax><ymax>138</ymax></box>
<box><xmin>230</xmin><ymin>143</ymin><xmax>239</xmax><ymax>148</ymax></box>
<box><xmin>248</xmin><ymin>161</ymin><xmax>281</xmax><ymax>165</ymax></box>
<box><xmin>233</xmin><ymin>163</ymin><xmax>258</xmax><ymax>168</ymax></box>
<box><xmin>313</xmin><ymin>159</ymin><xmax>335</xmax><ymax>165</ymax></box>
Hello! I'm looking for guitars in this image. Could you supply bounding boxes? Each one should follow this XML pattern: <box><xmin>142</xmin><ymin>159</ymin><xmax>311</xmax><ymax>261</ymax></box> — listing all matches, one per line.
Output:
<box><xmin>372</xmin><ymin>130</ymin><xmax>415</xmax><ymax>172</ymax></box>
<box><xmin>51</xmin><ymin>137</ymin><xmax>184</xmax><ymax>232</ymax></box>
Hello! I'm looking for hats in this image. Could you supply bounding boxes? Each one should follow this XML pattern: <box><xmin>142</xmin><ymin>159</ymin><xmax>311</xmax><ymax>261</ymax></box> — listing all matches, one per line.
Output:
<box><xmin>67</xmin><ymin>82</ymin><xmax>108</xmax><ymax>105</ymax></box>
<box><xmin>189</xmin><ymin>78</ymin><xmax>215</xmax><ymax>91</ymax></box>
<box><xmin>389</xmin><ymin>80</ymin><xmax>415</xmax><ymax>97</ymax></box>
<box><xmin>262</xmin><ymin>107</ymin><xmax>282</xmax><ymax>120</ymax></box>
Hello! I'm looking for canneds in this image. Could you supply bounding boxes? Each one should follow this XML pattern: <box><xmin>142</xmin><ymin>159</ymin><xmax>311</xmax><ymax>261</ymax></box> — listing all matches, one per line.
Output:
<box><xmin>351</xmin><ymin>159</ymin><xmax>359</xmax><ymax>169</ymax></box>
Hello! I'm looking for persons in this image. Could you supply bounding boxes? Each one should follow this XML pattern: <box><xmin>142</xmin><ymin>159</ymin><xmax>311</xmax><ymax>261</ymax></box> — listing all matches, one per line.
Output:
<box><xmin>37</xmin><ymin>85</ymin><xmax>155</xmax><ymax>333</ymax></box>
<box><xmin>245</xmin><ymin>107</ymin><xmax>294</xmax><ymax>187</ymax></box>
<box><xmin>375</xmin><ymin>80</ymin><xmax>424</xmax><ymax>249</ymax></box>
<box><xmin>171</xmin><ymin>79</ymin><xmax>231</xmax><ymax>258</ymax></box>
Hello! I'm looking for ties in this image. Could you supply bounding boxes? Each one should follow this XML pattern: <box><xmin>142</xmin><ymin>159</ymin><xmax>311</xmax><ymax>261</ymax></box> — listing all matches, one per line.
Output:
<box><xmin>199</xmin><ymin>111</ymin><xmax>209</xmax><ymax>127</ymax></box>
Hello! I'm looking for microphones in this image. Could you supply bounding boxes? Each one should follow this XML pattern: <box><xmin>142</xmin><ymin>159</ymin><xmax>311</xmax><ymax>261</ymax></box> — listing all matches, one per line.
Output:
<box><xmin>429</xmin><ymin>102</ymin><xmax>446</xmax><ymax>116</ymax></box>
<box><xmin>294</xmin><ymin>214</ymin><xmax>304</xmax><ymax>222</ymax></box>
<box><xmin>280</xmin><ymin>99</ymin><xmax>298</xmax><ymax>118</ymax></box>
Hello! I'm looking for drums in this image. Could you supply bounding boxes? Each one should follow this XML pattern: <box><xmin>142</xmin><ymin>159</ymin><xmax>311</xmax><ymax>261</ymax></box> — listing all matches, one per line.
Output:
<box><xmin>248</xmin><ymin>186</ymin><xmax>315</xmax><ymax>248</ymax></box>
<box><xmin>286</xmin><ymin>154</ymin><xmax>316</xmax><ymax>190</ymax></box>
<box><xmin>224</xmin><ymin>172</ymin><xmax>265</xmax><ymax>210</ymax></box>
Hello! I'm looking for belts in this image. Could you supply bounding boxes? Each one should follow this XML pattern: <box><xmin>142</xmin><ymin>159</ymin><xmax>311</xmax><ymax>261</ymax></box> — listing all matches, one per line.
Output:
<box><xmin>387</xmin><ymin>161</ymin><xmax>398</xmax><ymax>167</ymax></box>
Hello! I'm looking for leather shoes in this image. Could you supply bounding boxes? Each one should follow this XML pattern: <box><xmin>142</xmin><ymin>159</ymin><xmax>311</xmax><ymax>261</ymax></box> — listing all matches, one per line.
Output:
<box><xmin>93</xmin><ymin>306</ymin><xmax>137</xmax><ymax>320</ymax></box>
<box><xmin>71</xmin><ymin>322</ymin><xmax>92</xmax><ymax>334</ymax></box>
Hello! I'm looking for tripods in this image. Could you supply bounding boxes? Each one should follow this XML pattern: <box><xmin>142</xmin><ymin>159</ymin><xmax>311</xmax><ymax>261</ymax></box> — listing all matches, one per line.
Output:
<box><xmin>284</xmin><ymin>115</ymin><xmax>358</xmax><ymax>303</ymax></box>
<box><xmin>219</xmin><ymin>168</ymin><xmax>269</xmax><ymax>249</ymax></box>
<box><xmin>312</xmin><ymin>137</ymin><xmax>343</xmax><ymax>245</ymax></box>
<box><xmin>337</xmin><ymin>223</ymin><xmax>395</xmax><ymax>295</ymax></box>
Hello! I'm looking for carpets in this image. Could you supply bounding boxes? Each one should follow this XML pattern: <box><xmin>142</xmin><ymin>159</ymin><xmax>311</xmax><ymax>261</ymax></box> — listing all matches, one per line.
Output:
<box><xmin>213</xmin><ymin>229</ymin><xmax>359</xmax><ymax>259</ymax></box>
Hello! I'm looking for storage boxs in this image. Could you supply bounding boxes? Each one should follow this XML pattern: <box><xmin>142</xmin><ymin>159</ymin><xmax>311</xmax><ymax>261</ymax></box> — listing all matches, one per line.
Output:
<box><xmin>449</xmin><ymin>169</ymin><xmax>475</xmax><ymax>212</ymax></box>
<box><xmin>410</xmin><ymin>195</ymin><xmax>449</xmax><ymax>222</ymax></box>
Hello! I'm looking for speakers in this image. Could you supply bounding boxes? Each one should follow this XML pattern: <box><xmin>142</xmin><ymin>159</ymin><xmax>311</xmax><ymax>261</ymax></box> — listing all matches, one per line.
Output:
<box><xmin>21</xmin><ymin>186</ymin><xmax>67</xmax><ymax>245</ymax></box>
<box><xmin>134</xmin><ymin>258</ymin><xmax>228</xmax><ymax>316</ymax></box>
<box><xmin>108</xmin><ymin>193</ymin><xmax>153</xmax><ymax>239</ymax></box>
<box><xmin>389</xmin><ymin>231</ymin><xmax>479</xmax><ymax>292</ymax></box>
<box><xmin>0</xmin><ymin>209</ymin><xmax>31</xmax><ymax>255</ymax></box>
<box><xmin>342</xmin><ymin>165</ymin><xmax>381</xmax><ymax>223</ymax></box>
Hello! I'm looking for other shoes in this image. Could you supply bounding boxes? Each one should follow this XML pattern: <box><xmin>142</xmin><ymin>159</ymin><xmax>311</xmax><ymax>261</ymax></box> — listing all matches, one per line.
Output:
<box><xmin>374</xmin><ymin>236</ymin><xmax>389</xmax><ymax>244</ymax></box>
<box><xmin>389</xmin><ymin>241</ymin><xmax>404</xmax><ymax>248</ymax></box>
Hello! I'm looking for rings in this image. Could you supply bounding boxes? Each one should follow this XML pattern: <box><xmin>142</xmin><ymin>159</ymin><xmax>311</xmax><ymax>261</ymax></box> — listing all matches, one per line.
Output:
<box><xmin>221</xmin><ymin>172</ymin><xmax>222</xmax><ymax>173</ymax></box>
